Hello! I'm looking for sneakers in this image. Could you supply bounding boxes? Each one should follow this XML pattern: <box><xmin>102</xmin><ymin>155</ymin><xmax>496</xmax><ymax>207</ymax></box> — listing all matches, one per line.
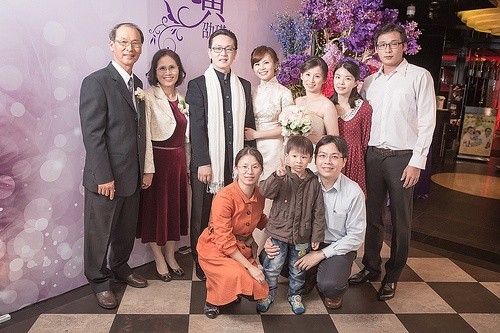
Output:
<box><xmin>256</xmin><ymin>295</ymin><xmax>273</xmax><ymax>312</ymax></box>
<box><xmin>288</xmin><ymin>295</ymin><xmax>305</xmax><ymax>314</ymax></box>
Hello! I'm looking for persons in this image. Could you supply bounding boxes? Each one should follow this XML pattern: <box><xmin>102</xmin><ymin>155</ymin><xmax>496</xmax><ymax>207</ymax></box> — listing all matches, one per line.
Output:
<box><xmin>260</xmin><ymin>135</ymin><xmax>366</xmax><ymax>308</ymax></box>
<box><xmin>256</xmin><ymin>135</ymin><xmax>325</xmax><ymax>314</ymax></box>
<box><xmin>348</xmin><ymin>23</ymin><xmax>436</xmax><ymax>300</ymax></box>
<box><xmin>136</xmin><ymin>48</ymin><xmax>191</xmax><ymax>283</ymax></box>
<box><xmin>185</xmin><ymin>29</ymin><xmax>256</xmax><ymax>283</ymax></box>
<box><xmin>463</xmin><ymin>126</ymin><xmax>492</xmax><ymax>149</ymax></box>
<box><xmin>244</xmin><ymin>45</ymin><xmax>294</xmax><ymax>270</ymax></box>
<box><xmin>196</xmin><ymin>148</ymin><xmax>270</xmax><ymax>319</ymax></box>
<box><xmin>329</xmin><ymin>61</ymin><xmax>373</xmax><ymax>199</ymax></box>
<box><xmin>294</xmin><ymin>56</ymin><xmax>339</xmax><ymax>172</ymax></box>
<box><xmin>79</xmin><ymin>22</ymin><xmax>147</xmax><ymax>309</ymax></box>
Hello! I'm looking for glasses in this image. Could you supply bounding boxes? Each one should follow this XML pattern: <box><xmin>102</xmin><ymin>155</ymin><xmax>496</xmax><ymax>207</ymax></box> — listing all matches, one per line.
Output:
<box><xmin>316</xmin><ymin>154</ymin><xmax>345</xmax><ymax>161</ymax></box>
<box><xmin>211</xmin><ymin>47</ymin><xmax>236</xmax><ymax>53</ymax></box>
<box><xmin>156</xmin><ymin>65</ymin><xmax>179</xmax><ymax>72</ymax></box>
<box><xmin>377</xmin><ymin>41</ymin><xmax>402</xmax><ymax>50</ymax></box>
<box><xmin>114</xmin><ymin>40</ymin><xmax>142</xmax><ymax>48</ymax></box>
<box><xmin>236</xmin><ymin>165</ymin><xmax>261</xmax><ymax>172</ymax></box>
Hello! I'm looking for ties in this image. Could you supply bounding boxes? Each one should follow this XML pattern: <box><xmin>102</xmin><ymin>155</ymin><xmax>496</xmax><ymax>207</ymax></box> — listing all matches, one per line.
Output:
<box><xmin>128</xmin><ymin>77</ymin><xmax>135</xmax><ymax>100</ymax></box>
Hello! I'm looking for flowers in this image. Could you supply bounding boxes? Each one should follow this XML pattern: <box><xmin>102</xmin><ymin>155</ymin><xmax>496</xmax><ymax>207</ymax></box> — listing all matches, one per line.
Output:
<box><xmin>177</xmin><ymin>97</ymin><xmax>190</xmax><ymax>115</ymax></box>
<box><xmin>134</xmin><ymin>86</ymin><xmax>147</xmax><ymax>102</ymax></box>
<box><xmin>267</xmin><ymin>0</ymin><xmax>422</xmax><ymax>97</ymax></box>
<box><xmin>276</xmin><ymin>104</ymin><xmax>314</xmax><ymax>141</ymax></box>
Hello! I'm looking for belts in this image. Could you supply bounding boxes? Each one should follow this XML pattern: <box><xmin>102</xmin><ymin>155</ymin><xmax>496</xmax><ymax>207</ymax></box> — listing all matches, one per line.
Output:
<box><xmin>368</xmin><ymin>147</ymin><xmax>411</xmax><ymax>157</ymax></box>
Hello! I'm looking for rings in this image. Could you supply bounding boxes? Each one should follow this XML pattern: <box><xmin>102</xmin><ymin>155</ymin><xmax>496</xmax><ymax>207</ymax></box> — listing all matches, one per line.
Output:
<box><xmin>143</xmin><ymin>185</ymin><xmax>144</xmax><ymax>187</ymax></box>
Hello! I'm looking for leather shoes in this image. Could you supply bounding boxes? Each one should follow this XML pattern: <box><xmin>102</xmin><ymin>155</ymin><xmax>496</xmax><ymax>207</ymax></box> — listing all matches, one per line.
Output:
<box><xmin>195</xmin><ymin>263</ymin><xmax>207</xmax><ymax>281</ymax></box>
<box><xmin>165</xmin><ymin>260</ymin><xmax>184</xmax><ymax>277</ymax></box>
<box><xmin>204</xmin><ymin>302</ymin><xmax>220</xmax><ymax>319</ymax></box>
<box><xmin>299</xmin><ymin>279</ymin><xmax>315</xmax><ymax>294</ymax></box>
<box><xmin>377</xmin><ymin>282</ymin><xmax>397</xmax><ymax>300</ymax></box>
<box><xmin>95</xmin><ymin>290</ymin><xmax>118</xmax><ymax>309</ymax></box>
<box><xmin>348</xmin><ymin>267</ymin><xmax>381</xmax><ymax>284</ymax></box>
<box><xmin>112</xmin><ymin>272</ymin><xmax>148</xmax><ymax>287</ymax></box>
<box><xmin>155</xmin><ymin>264</ymin><xmax>172</xmax><ymax>282</ymax></box>
<box><xmin>325</xmin><ymin>296</ymin><xmax>343</xmax><ymax>308</ymax></box>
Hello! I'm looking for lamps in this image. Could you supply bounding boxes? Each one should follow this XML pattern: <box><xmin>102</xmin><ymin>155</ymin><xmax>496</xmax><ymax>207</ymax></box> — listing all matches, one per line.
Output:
<box><xmin>457</xmin><ymin>0</ymin><xmax>500</xmax><ymax>36</ymax></box>
